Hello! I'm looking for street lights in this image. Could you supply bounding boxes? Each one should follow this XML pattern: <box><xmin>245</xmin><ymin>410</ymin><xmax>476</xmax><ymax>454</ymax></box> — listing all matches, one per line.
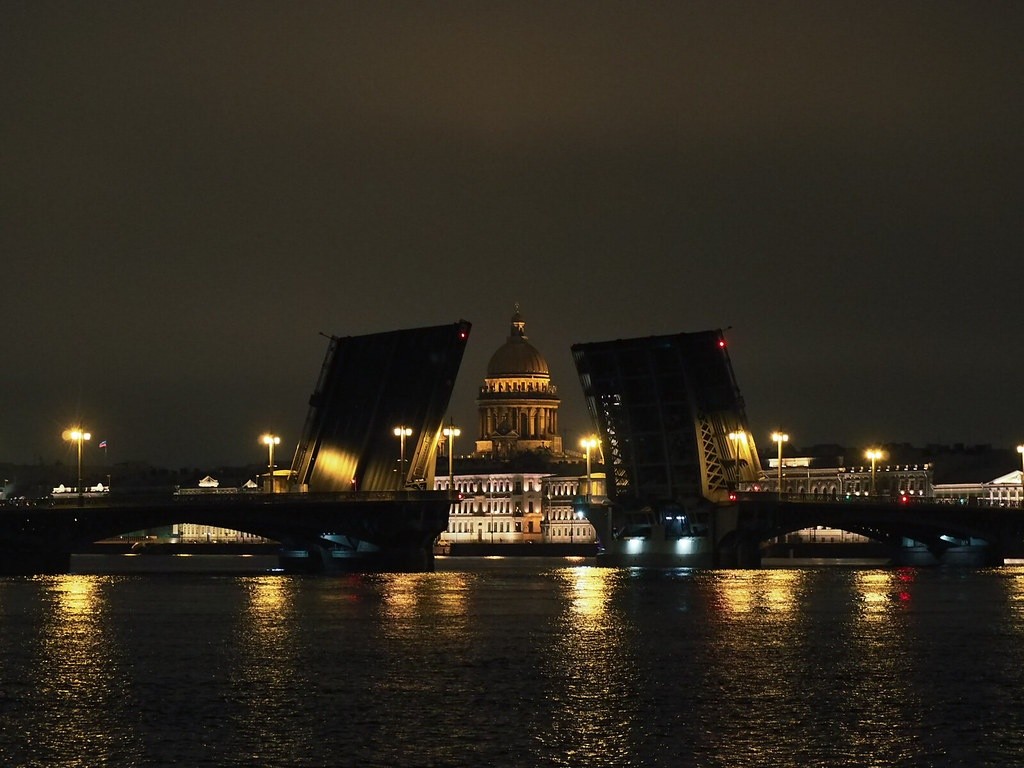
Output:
<box><xmin>443</xmin><ymin>417</ymin><xmax>460</xmax><ymax>491</ymax></box>
<box><xmin>393</xmin><ymin>424</ymin><xmax>413</xmax><ymax>490</ymax></box>
<box><xmin>772</xmin><ymin>430</ymin><xmax>789</xmax><ymax>503</ymax></box>
<box><xmin>728</xmin><ymin>431</ymin><xmax>747</xmax><ymax>490</ymax></box>
<box><xmin>582</xmin><ymin>439</ymin><xmax>597</xmax><ymax>502</ymax></box>
<box><xmin>865</xmin><ymin>450</ymin><xmax>883</xmax><ymax>496</ymax></box>
<box><xmin>260</xmin><ymin>432</ymin><xmax>280</xmax><ymax>491</ymax></box>
<box><xmin>65</xmin><ymin>428</ymin><xmax>91</xmax><ymax>509</ymax></box>
<box><xmin>1016</xmin><ymin>445</ymin><xmax>1024</xmax><ymax>480</ymax></box>
